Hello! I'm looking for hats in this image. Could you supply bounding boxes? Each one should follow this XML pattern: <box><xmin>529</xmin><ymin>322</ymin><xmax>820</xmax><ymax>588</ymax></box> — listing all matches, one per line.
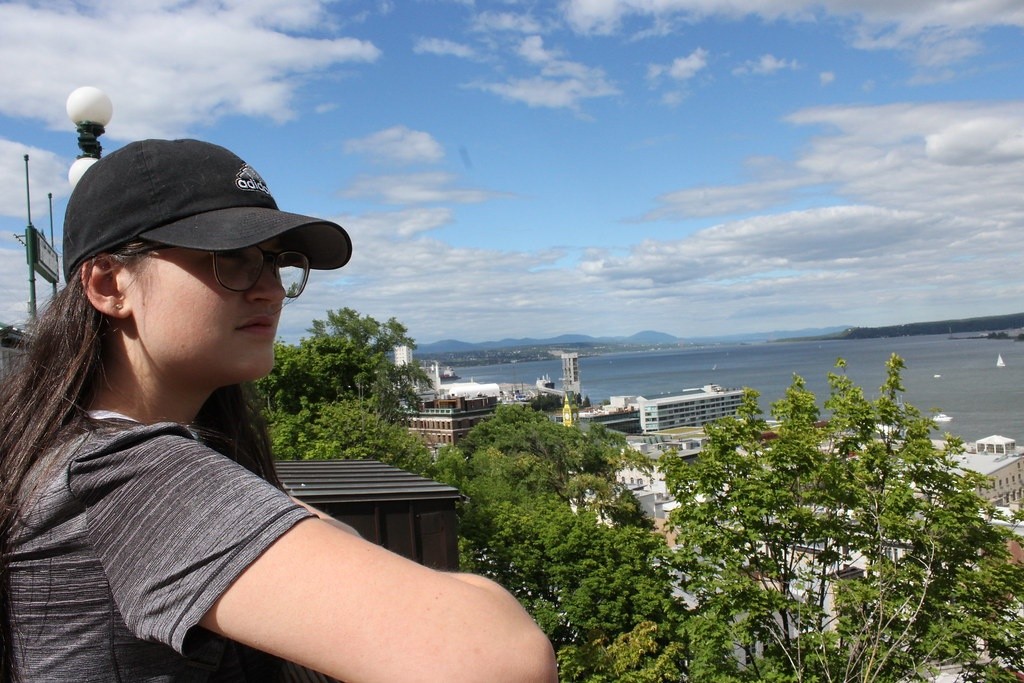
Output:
<box><xmin>62</xmin><ymin>138</ymin><xmax>353</xmax><ymax>284</ymax></box>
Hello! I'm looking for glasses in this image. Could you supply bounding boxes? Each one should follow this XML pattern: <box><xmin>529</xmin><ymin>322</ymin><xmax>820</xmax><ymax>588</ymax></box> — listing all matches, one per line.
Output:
<box><xmin>113</xmin><ymin>241</ymin><xmax>310</xmax><ymax>298</ymax></box>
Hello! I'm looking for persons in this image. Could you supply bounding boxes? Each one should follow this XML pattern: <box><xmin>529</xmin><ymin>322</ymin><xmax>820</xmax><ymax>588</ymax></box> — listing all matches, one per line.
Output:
<box><xmin>1</xmin><ymin>140</ymin><xmax>560</xmax><ymax>683</ymax></box>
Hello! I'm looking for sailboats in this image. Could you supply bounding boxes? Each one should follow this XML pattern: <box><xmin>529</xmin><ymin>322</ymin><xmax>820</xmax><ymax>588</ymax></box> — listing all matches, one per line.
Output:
<box><xmin>997</xmin><ymin>354</ymin><xmax>1006</xmax><ymax>367</ymax></box>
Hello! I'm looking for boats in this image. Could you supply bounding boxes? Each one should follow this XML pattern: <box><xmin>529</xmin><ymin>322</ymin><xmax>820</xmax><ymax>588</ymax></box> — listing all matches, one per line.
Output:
<box><xmin>439</xmin><ymin>366</ymin><xmax>461</xmax><ymax>380</ymax></box>
<box><xmin>933</xmin><ymin>413</ymin><xmax>953</xmax><ymax>421</ymax></box>
